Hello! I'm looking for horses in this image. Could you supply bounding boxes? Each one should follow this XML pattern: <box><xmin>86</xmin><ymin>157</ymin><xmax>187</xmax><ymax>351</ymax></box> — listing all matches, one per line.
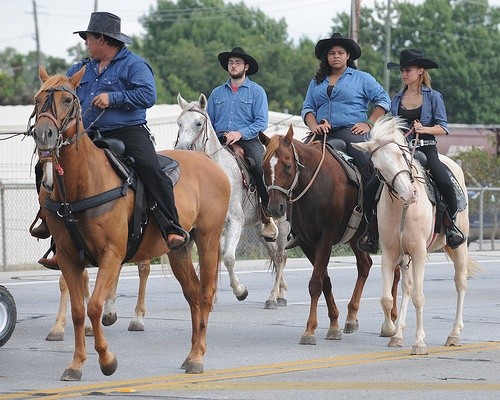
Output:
<box><xmin>351</xmin><ymin>115</ymin><xmax>485</xmax><ymax>355</ymax></box>
<box><xmin>258</xmin><ymin>123</ymin><xmax>401</xmax><ymax>345</ymax></box>
<box><xmin>174</xmin><ymin>93</ymin><xmax>291</xmax><ymax>308</ymax></box>
<box><xmin>34</xmin><ymin>64</ymin><xmax>230</xmax><ymax>381</ymax></box>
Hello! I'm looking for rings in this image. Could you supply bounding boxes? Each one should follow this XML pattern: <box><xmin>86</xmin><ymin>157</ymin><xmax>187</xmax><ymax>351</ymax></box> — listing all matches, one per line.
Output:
<box><xmin>98</xmin><ymin>104</ymin><xmax>101</xmax><ymax>106</ymax></box>
<box><xmin>230</xmin><ymin>139</ymin><xmax>233</xmax><ymax>141</ymax></box>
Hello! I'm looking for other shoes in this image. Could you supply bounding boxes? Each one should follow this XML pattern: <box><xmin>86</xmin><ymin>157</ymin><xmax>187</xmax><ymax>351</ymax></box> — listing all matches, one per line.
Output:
<box><xmin>284</xmin><ymin>237</ymin><xmax>299</xmax><ymax>249</ymax></box>
<box><xmin>31</xmin><ymin>219</ymin><xmax>51</xmax><ymax>239</ymax></box>
<box><xmin>446</xmin><ymin>225</ymin><xmax>462</xmax><ymax>249</ymax></box>
<box><xmin>357</xmin><ymin>236</ymin><xmax>379</xmax><ymax>252</ymax></box>
<box><xmin>260</xmin><ymin>223</ymin><xmax>276</xmax><ymax>238</ymax></box>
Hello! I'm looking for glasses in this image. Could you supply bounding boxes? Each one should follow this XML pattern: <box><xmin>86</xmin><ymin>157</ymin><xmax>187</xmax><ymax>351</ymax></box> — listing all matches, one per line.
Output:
<box><xmin>227</xmin><ymin>61</ymin><xmax>245</xmax><ymax>66</ymax></box>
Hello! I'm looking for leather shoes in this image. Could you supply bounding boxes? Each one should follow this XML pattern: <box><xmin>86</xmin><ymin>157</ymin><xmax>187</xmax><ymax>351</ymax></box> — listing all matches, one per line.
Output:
<box><xmin>168</xmin><ymin>234</ymin><xmax>184</xmax><ymax>249</ymax></box>
<box><xmin>38</xmin><ymin>254</ymin><xmax>58</xmax><ymax>268</ymax></box>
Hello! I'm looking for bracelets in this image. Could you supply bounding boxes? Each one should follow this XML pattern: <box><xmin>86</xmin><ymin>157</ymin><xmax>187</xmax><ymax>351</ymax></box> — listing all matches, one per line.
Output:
<box><xmin>365</xmin><ymin>122</ymin><xmax>372</xmax><ymax>130</ymax></box>
<box><xmin>366</xmin><ymin>119</ymin><xmax>374</xmax><ymax>126</ymax></box>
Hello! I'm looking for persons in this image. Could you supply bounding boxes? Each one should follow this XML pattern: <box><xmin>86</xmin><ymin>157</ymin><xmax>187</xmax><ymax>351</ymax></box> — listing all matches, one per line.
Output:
<box><xmin>358</xmin><ymin>48</ymin><xmax>465</xmax><ymax>254</ymax></box>
<box><xmin>205</xmin><ymin>47</ymin><xmax>277</xmax><ymax>239</ymax></box>
<box><xmin>285</xmin><ymin>33</ymin><xmax>391</xmax><ymax>254</ymax></box>
<box><xmin>31</xmin><ymin>12</ymin><xmax>185</xmax><ymax>270</ymax></box>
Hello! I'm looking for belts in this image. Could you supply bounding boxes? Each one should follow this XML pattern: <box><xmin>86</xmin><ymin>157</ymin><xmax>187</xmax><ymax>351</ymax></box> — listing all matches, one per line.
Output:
<box><xmin>409</xmin><ymin>139</ymin><xmax>436</xmax><ymax>148</ymax></box>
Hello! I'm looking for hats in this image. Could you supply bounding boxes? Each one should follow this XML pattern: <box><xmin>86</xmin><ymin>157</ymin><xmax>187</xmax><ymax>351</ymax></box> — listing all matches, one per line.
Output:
<box><xmin>387</xmin><ymin>49</ymin><xmax>439</xmax><ymax>70</ymax></box>
<box><xmin>73</xmin><ymin>12</ymin><xmax>132</xmax><ymax>44</ymax></box>
<box><xmin>218</xmin><ymin>47</ymin><xmax>259</xmax><ymax>75</ymax></box>
<box><xmin>315</xmin><ymin>33</ymin><xmax>361</xmax><ymax>61</ymax></box>
